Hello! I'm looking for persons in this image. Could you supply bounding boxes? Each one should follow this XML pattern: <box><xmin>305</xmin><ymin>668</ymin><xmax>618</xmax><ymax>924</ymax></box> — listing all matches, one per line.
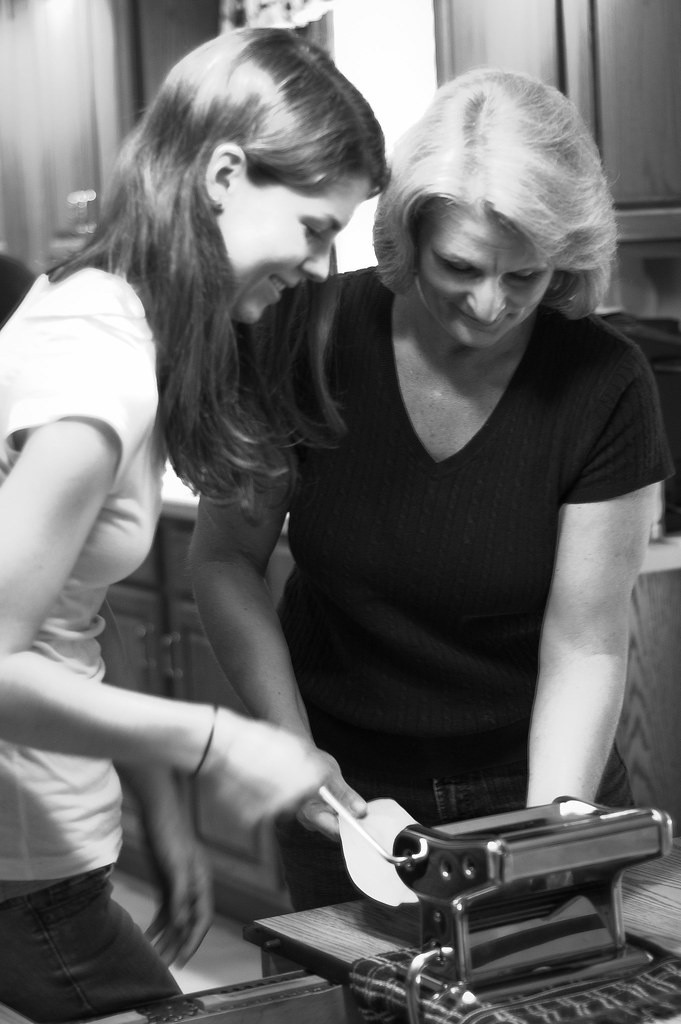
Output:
<box><xmin>0</xmin><ymin>24</ymin><xmax>387</xmax><ymax>1024</ymax></box>
<box><xmin>190</xmin><ymin>70</ymin><xmax>676</xmax><ymax>911</ymax></box>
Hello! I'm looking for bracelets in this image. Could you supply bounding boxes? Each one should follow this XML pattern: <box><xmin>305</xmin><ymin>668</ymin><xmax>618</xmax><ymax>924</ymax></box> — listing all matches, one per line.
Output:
<box><xmin>193</xmin><ymin>704</ymin><xmax>217</xmax><ymax>777</ymax></box>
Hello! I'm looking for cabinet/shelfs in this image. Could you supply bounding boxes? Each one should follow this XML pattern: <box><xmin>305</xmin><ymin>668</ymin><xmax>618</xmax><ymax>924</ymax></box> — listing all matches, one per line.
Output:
<box><xmin>100</xmin><ymin>518</ymin><xmax>296</xmax><ymax>911</ymax></box>
<box><xmin>433</xmin><ymin>0</ymin><xmax>681</xmax><ymax>243</ymax></box>
